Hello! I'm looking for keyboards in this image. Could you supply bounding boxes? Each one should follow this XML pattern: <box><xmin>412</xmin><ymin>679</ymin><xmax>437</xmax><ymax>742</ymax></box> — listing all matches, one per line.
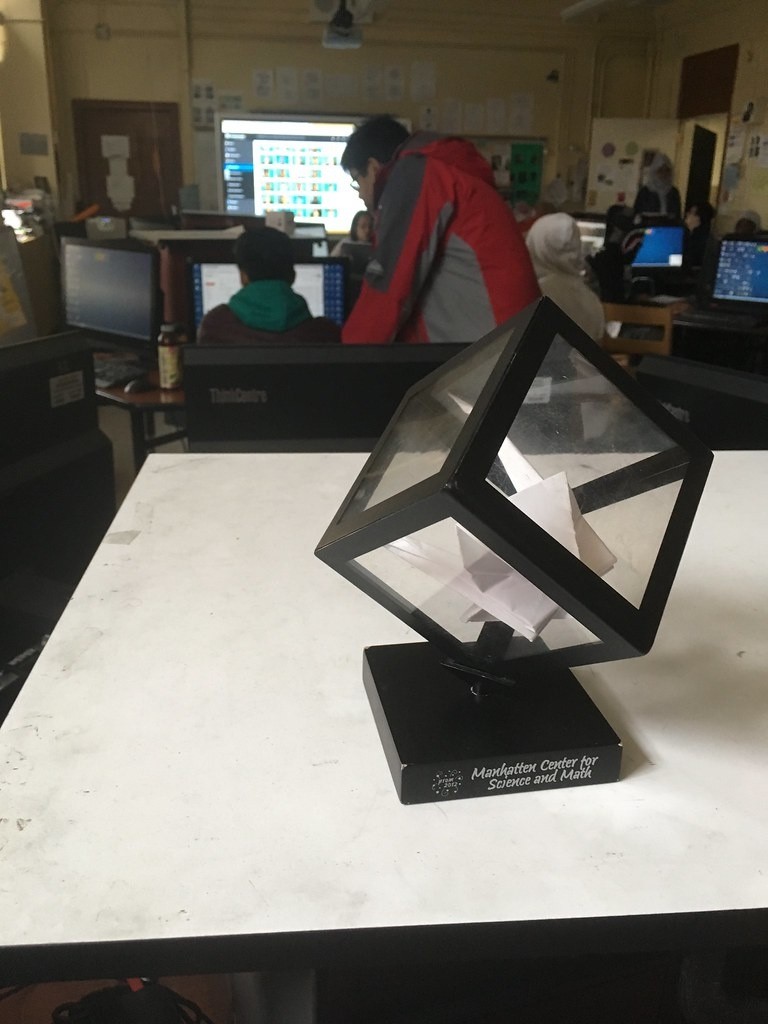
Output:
<box><xmin>58</xmin><ymin>348</ymin><xmax>148</xmax><ymax>388</ymax></box>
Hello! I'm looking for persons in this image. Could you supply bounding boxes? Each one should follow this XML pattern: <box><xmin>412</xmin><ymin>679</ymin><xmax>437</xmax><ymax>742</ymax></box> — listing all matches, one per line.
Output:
<box><xmin>331</xmin><ymin>210</ymin><xmax>372</xmax><ymax>257</ymax></box>
<box><xmin>589</xmin><ymin>201</ymin><xmax>721</xmax><ymax>299</ymax></box>
<box><xmin>340</xmin><ymin>117</ymin><xmax>537</xmax><ymax>346</ymax></box>
<box><xmin>633</xmin><ymin>153</ymin><xmax>681</xmax><ymax>216</ymax></box>
<box><xmin>196</xmin><ymin>226</ymin><xmax>341</xmax><ymax>344</ymax></box>
<box><xmin>526</xmin><ymin>211</ymin><xmax>605</xmax><ymax>340</ymax></box>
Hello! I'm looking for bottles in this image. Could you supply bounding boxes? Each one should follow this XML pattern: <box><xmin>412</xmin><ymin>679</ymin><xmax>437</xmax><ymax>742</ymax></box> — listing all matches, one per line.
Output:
<box><xmin>157</xmin><ymin>324</ymin><xmax>183</xmax><ymax>391</ymax></box>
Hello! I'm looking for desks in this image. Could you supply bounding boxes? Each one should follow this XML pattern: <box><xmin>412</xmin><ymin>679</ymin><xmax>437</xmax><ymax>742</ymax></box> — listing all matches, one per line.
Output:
<box><xmin>1</xmin><ymin>299</ymin><xmax>768</xmax><ymax>1024</ymax></box>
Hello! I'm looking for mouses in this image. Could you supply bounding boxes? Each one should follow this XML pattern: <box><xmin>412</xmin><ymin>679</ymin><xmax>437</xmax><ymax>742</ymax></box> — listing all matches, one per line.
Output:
<box><xmin>123</xmin><ymin>379</ymin><xmax>146</xmax><ymax>394</ymax></box>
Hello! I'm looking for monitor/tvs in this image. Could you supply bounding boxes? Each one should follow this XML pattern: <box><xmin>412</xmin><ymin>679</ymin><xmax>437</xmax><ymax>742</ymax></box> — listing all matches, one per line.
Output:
<box><xmin>566</xmin><ymin>213</ymin><xmax>767</xmax><ymax>324</ymax></box>
<box><xmin>60</xmin><ymin>109</ymin><xmax>412</xmax><ymax>351</ymax></box>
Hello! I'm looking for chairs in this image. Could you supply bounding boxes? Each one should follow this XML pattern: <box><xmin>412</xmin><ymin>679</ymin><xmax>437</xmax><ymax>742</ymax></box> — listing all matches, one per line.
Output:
<box><xmin>180</xmin><ymin>343</ymin><xmax>468</xmax><ymax>453</ymax></box>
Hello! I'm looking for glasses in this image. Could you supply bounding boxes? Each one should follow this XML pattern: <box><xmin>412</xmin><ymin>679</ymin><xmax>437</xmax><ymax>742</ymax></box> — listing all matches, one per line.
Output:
<box><xmin>350</xmin><ymin>170</ymin><xmax>365</xmax><ymax>190</ymax></box>
